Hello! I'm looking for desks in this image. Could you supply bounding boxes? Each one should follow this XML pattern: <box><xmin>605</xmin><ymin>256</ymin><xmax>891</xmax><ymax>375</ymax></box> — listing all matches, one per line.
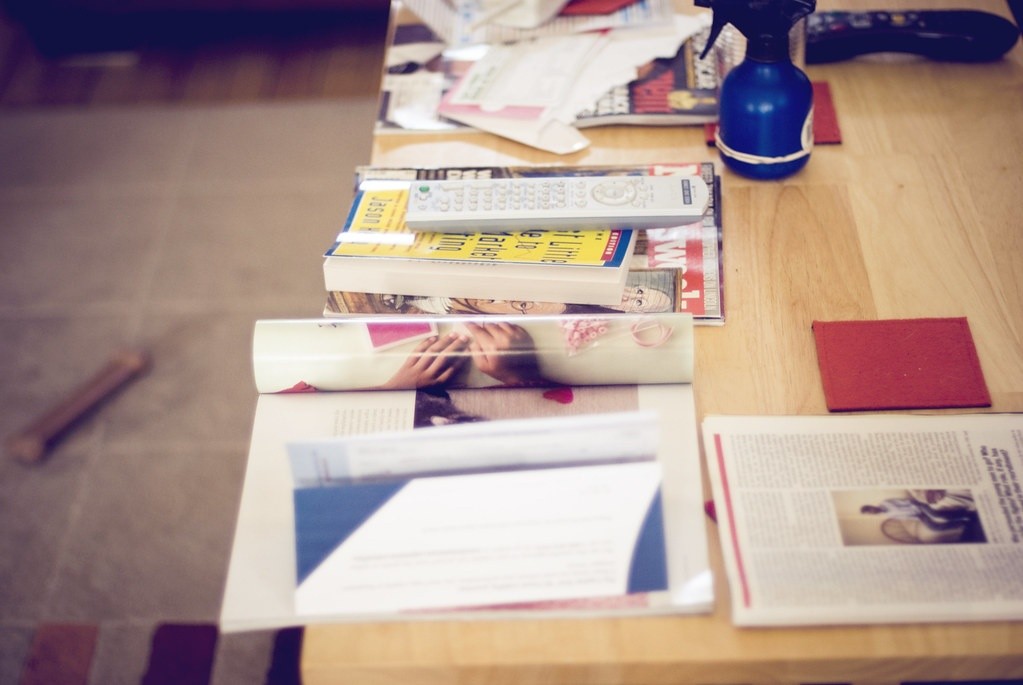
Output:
<box><xmin>298</xmin><ymin>0</ymin><xmax>1023</xmax><ymax>685</ymax></box>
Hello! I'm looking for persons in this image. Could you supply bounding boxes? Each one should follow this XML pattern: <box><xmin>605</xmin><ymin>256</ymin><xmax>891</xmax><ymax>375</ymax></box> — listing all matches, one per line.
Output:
<box><xmin>860</xmin><ymin>499</ymin><xmax>968</xmax><ymax>533</ymax></box>
<box><xmin>383</xmin><ymin>323</ymin><xmax>545</xmax><ymax>392</ymax></box>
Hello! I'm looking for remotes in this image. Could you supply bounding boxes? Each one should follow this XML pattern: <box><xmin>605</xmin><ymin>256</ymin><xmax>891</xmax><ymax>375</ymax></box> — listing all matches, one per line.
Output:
<box><xmin>805</xmin><ymin>9</ymin><xmax>1020</xmax><ymax>64</ymax></box>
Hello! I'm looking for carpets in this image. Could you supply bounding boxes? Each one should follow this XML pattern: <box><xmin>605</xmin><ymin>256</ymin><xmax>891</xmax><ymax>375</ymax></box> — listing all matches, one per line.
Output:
<box><xmin>0</xmin><ymin>617</ymin><xmax>300</xmax><ymax>685</ymax></box>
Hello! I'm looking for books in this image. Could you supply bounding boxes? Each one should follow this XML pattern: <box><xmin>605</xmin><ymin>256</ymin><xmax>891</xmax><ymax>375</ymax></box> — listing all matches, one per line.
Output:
<box><xmin>217</xmin><ymin>0</ymin><xmax>1023</xmax><ymax>633</ymax></box>
